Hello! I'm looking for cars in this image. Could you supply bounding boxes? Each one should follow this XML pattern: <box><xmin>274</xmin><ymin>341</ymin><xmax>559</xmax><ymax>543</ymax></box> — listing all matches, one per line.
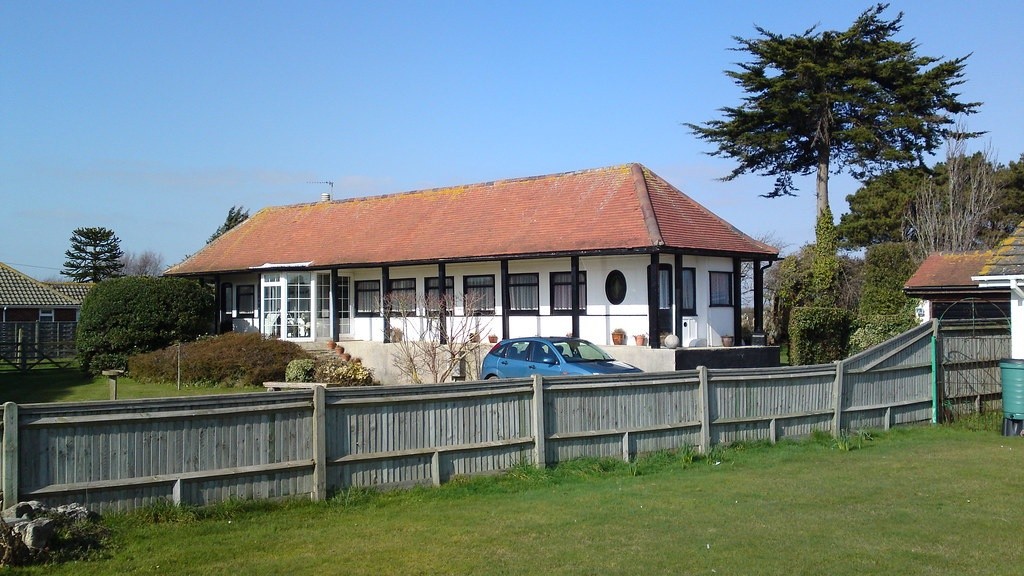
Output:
<box><xmin>479</xmin><ymin>336</ymin><xmax>648</xmax><ymax>380</ymax></box>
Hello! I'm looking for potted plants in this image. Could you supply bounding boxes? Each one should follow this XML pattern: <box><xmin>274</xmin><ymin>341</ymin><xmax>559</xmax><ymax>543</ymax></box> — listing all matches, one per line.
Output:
<box><xmin>489</xmin><ymin>335</ymin><xmax>497</xmax><ymax>342</ymax></box>
<box><xmin>335</xmin><ymin>344</ymin><xmax>343</xmax><ymax>355</ymax></box>
<box><xmin>612</xmin><ymin>329</ymin><xmax>625</xmax><ymax>345</ymax></box>
<box><xmin>341</xmin><ymin>353</ymin><xmax>351</xmax><ymax>361</ymax></box>
<box><xmin>720</xmin><ymin>333</ymin><xmax>733</xmax><ymax>348</ymax></box>
<box><xmin>633</xmin><ymin>334</ymin><xmax>646</xmax><ymax>345</ymax></box>
<box><xmin>390</xmin><ymin>327</ymin><xmax>402</xmax><ymax>343</ymax></box>
<box><xmin>328</xmin><ymin>340</ymin><xmax>337</xmax><ymax>349</ymax></box>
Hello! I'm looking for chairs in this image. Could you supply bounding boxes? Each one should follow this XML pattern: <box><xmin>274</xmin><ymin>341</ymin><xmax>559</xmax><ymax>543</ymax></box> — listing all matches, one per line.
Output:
<box><xmin>506</xmin><ymin>346</ymin><xmax>516</xmax><ymax>359</ymax></box>
<box><xmin>550</xmin><ymin>345</ymin><xmax>564</xmax><ymax>360</ymax></box>
<box><xmin>534</xmin><ymin>347</ymin><xmax>545</xmax><ymax>362</ymax></box>
